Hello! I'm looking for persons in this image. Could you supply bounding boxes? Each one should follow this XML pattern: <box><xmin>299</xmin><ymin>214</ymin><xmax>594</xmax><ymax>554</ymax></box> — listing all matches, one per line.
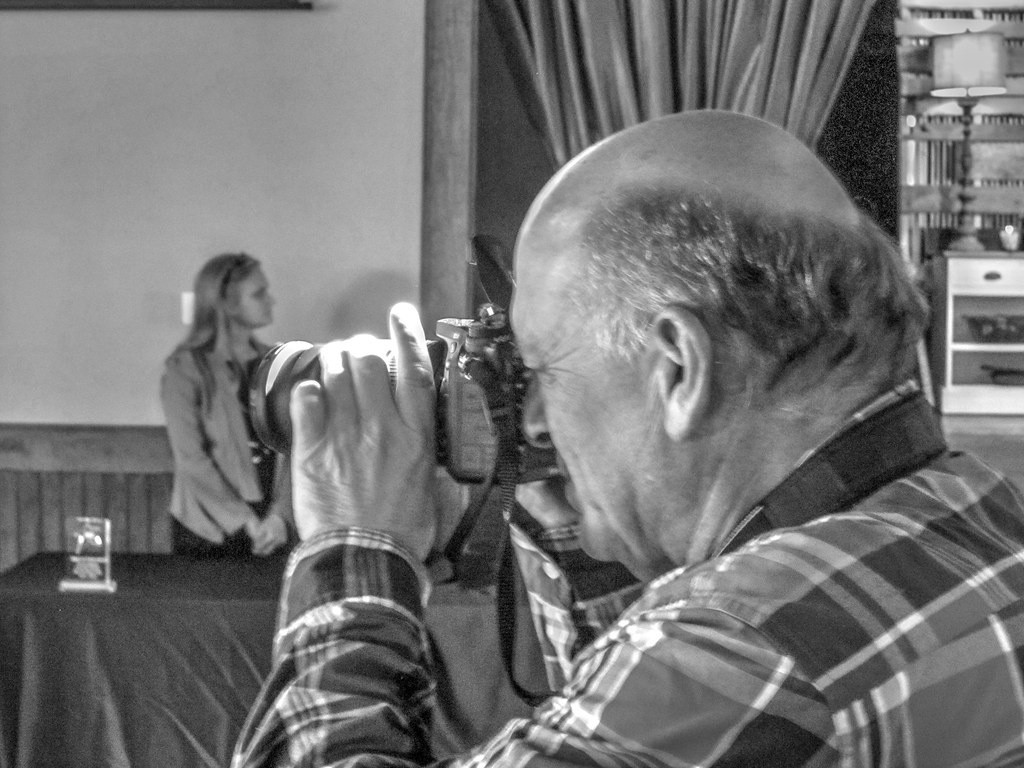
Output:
<box><xmin>161</xmin><ymin>253</ymin><xmax>297</xmax><ymax>557</ymax></box>
<box><xmin>232</xmin><ymin>110</ymin><xmax>1024</xmax><ymax>768</ymax></box>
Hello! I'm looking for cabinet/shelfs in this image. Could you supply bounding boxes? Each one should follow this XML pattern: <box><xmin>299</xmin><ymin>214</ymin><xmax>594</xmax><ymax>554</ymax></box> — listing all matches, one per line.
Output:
<box><xmin>931</xmin><ymin>250</ymin><xmax>1024</xmax><ymax>414</ymax></box>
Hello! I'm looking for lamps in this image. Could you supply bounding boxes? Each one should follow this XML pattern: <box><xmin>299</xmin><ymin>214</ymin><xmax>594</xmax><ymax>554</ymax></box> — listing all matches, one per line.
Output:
<box><xmin>932</xmin><ymin>31</ymin><xmax>1009</xmax><ymax>249</ymax></box>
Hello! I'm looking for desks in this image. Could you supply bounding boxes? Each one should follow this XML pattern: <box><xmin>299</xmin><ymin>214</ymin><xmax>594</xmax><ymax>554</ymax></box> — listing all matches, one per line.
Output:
<box><xmin>0</xmin><ymin>546</ymin><xmax>547</xmax><ymax>768</ymax></box>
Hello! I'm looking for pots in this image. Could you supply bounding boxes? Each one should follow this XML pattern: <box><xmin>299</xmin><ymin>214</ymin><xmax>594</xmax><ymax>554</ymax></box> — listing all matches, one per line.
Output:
<box><xmin>981</xmin><ymin>364</ymin><xmax>1024</xmax><ymax>385</ymax></box>
<box><xmin>961</xmin><ymin>315</ymin><xmax>1024</xmax><ymax>342</ymax></box>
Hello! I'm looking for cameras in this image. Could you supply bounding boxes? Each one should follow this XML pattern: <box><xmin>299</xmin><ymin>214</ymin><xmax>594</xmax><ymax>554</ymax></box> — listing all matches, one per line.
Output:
<box><xmin>248</xmin><ymin>238</ymin><xmax>561</xmax><ymax>487</ymax></box>
